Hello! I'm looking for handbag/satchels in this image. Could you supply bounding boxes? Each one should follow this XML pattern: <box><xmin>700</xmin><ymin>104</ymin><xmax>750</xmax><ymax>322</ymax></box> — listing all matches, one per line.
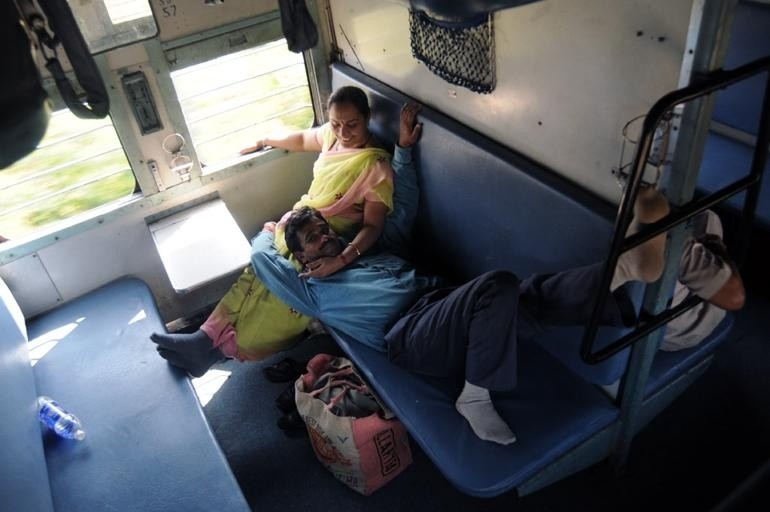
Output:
<box><xmin>295</xmin><ymin>354</ymin><xmax>413</xmax><ymax>497</ymax></box>
<box><xmin>2</xmin><ymin>0</ymin><xmax>109</xmax><ymax>170</ymax></box>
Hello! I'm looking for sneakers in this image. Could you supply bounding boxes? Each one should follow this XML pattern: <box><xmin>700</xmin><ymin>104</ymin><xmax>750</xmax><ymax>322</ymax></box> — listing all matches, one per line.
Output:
<box><xmin>262</xmin><ymin>357</ymin><xmax>302</xmax><ymax>384</ymax></box>
<box><xmin>276</xmin><ymin>375</ymin><xmax>298</xmax><ymax>413</ymax></box>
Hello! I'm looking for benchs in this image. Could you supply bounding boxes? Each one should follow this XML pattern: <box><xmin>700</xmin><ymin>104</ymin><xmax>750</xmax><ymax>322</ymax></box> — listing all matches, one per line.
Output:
<box><xmin>315</xmin><ymin>0</ymin><xmax>770</xmax><ymax>495</ymax></box>
<box><xmin>0</xmin><ymin>274</ymin><xmax>252</xmax><ymax>512</ymax></box>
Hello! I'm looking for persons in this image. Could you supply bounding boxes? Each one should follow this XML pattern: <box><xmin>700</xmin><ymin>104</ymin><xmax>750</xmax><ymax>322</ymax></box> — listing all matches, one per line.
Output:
<box><xmin>150</xmin><ymin>86</ymin><xmax>395</xmax><ymax>378</ymax></box>
<box><xmin>250</xmin><ymin>103</ymin><xmax>670</xmax><ymax>445</ymax></box>
<box><xmin>658</xmin><ymin>209</ymin><xmax>745</xmax><ymax>353</ymax></box>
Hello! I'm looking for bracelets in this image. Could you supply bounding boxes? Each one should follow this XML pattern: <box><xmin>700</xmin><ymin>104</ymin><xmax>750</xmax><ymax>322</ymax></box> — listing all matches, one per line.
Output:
<box><xmin>348</xmin><ymin>243</ymin><xmax>360</xmax><ymax>256</ymax></box>
<box><xmin>340</xmin><ymin>255</ymin><xmax>348</xmax><ymax>266</ymax></box>
<box><xmin>263</xmin><ymin>137</ymin><xmax>268</xmax><ymax>147</ymax></box>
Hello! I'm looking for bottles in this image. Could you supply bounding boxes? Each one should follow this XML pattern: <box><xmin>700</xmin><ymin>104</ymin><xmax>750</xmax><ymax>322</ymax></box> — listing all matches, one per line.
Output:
<box><xmin>38</xmin><ymin>396</ymin><xmax>86</xmax><ymax>442</ymax></box>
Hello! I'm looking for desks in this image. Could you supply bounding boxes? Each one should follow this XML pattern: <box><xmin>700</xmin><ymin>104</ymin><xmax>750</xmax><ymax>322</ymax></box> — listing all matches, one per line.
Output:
<box><xmin>149</xmin><ymin>198</ymin><xmax>254</xmax><ymax>294</ymax></box>
